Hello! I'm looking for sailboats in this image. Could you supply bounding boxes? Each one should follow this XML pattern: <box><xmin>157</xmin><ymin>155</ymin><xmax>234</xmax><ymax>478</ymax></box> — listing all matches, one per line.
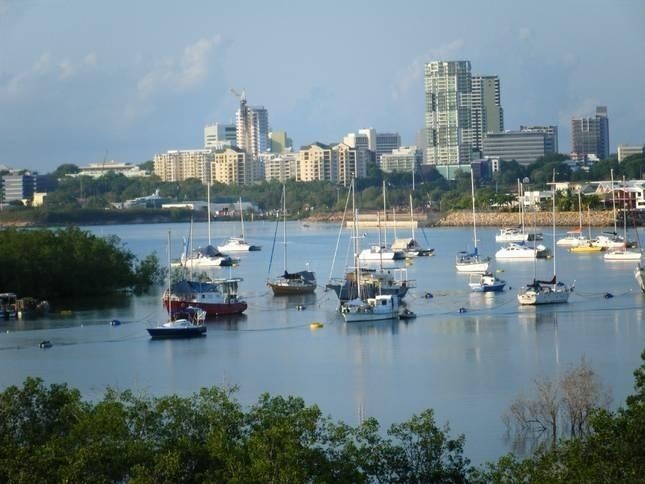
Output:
<box><xmin>266</xmin><ymin>186</ymin><xmax>318</xmax><ymax>296</ymax></box>
<box><xmin>323</xmin><ymin>174</ymin><xmax>434</xmax><ymax>324</ymax></box>
<box><xmin>455</xmin><ymin>169</ymin><xmax>645</xmax><ymax>305</ymax></box>
<box><xmin>145</xmin><ymin>180</ymin><xmax>261</xmax><ymax>340</ymax></box>
